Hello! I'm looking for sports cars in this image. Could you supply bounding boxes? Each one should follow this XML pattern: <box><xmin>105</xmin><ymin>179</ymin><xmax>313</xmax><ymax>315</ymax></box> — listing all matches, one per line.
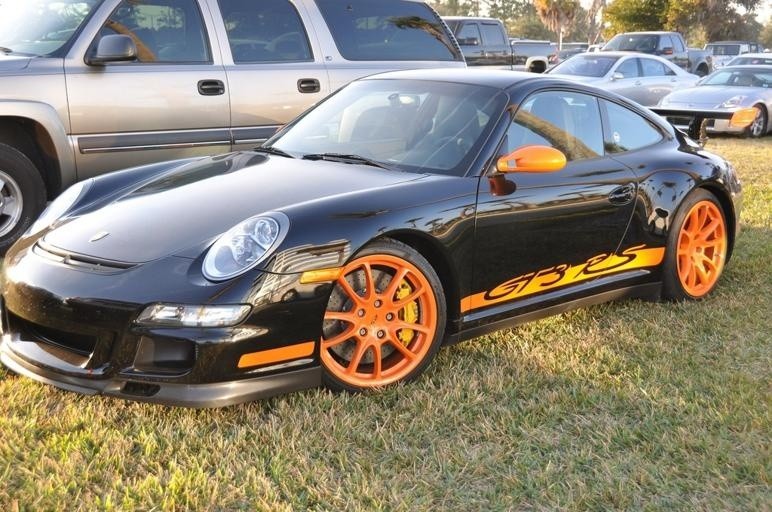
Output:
<box><xmin>656</xmin><ymin>64</ymin><xmax>772</xmax><ymax>140</ymax></box>
<box><xmin>0</xmin><ymin>67</ymin><xmax>741</xmax><ymax>410</ymax></box>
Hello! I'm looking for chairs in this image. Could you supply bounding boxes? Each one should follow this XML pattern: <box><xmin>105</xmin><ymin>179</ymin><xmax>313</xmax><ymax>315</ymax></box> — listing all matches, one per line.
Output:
<box><xmin>527</xmin><ymin>94</ymin><xmax>576</xmax><ymax>139</ymax></box>
<box><xmin>416</xmin><ymin>95</ymin><xmax>480</xmax><ymax>170</ymax></box>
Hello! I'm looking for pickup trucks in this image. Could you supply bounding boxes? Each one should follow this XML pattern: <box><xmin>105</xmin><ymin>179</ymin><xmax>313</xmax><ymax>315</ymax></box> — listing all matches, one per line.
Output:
<box><xmin>601</xmin><ymin>30</ymin><xmax>714</xmax><ymax>78</ymax></box>
<box><xmin>441</xmin><ymin>16</ymin><xmax>560</xmax><ymax>74</ymax></box>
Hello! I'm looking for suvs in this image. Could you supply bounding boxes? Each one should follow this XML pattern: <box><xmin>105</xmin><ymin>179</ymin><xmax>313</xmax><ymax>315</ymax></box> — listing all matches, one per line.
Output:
<box><xmin>1</xmin><ymin>1</ymin><xmax>471</xmax><ymax>253</ymax></box>
<box><xmin>702</xmin><ymin>41</ymin><xmax>751</xmax><ymax>72</ymax></box>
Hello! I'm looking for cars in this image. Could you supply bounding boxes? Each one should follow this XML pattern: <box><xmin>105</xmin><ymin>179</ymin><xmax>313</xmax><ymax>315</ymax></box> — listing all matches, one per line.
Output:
<box><xmin>540</xmin><ymin>50</ymin><xmax>703</xmax><ymax>108</ymax></box>
<box><xmin>560</xmin><ymin>43</ymin><xmax>606</xmax><ymax>60</ymax></box>
<box><xmin>749</xmin><ymin>42</ymin><xmax>766</xmax><ymax>64</ymax></box>
<box><xmin>722</xmin><ymin>53</ymin><xmax>771</xmax><ymax>65</ymax></box>
